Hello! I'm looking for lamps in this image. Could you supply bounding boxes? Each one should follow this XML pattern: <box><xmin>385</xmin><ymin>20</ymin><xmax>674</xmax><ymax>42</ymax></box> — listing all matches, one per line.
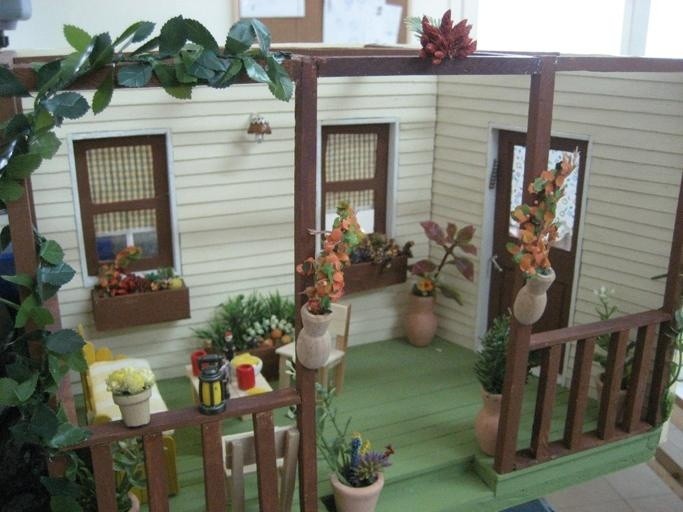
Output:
<box><xmin>246</xmin><ymin>113</ymin><xmax>272</xmax><ymax>144</ymax></box>
<box><xmin>192</xmin><ymin>355</ymin><xmax>233</xmax><ymax>416</ymax></box>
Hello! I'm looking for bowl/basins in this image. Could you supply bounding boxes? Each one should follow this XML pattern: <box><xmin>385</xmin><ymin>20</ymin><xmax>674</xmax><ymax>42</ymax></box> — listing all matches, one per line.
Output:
<box><xmin>230</xmin><ymin>355</ymin><xmax>263</xmax><ymax>378</ymax></box>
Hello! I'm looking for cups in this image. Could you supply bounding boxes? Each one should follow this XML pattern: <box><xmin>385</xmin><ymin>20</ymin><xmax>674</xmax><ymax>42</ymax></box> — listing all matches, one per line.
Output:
<box><xmin>192</xmin><ymin>350</ymin><xmax>254</xmax><ymax>390</ymax></box>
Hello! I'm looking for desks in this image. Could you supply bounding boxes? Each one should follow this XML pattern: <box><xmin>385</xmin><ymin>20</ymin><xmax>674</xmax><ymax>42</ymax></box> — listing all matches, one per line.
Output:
<box><xmin>186</xmin><ymin>358</ymin><xmax>274</xmax><ymax>423</ymax></box>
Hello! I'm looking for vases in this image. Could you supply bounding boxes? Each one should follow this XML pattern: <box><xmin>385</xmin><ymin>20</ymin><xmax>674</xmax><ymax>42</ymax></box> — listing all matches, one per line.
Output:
<box><xmin>317</xmin><ymin>249</ymin><xmax>407</xmax><ymax>299</ymax></box>
<box><xmin>295</xmin><ymin>303</ymin><xmax>333</xmax><ymax>370</ymax></box>
<box><xmin>112</xmin><ymin>387</ymin><xmax>154</xmax><ymax>427</ymax></box>
<box><xmin>90</xmin><ymin>279</ymin><xmax>191</xmax><ymax>332</ymax></box>
<box><xmin>331</xmin><ymin>468</ymin><xmax>383</xmax><ymax>511</ymax></box>
<box><xmin>595</xmin><ymin>373</ymin><xmax>633</xmax><ymax>423</ymax></box>
<box><xmin>405</xmin><ymin>292</ymin><xmax>437</xmax><ymax>348</ymax></box>
<box><xmin>232</xmin><ymin>347</ymin><xmax>277</xmax><ymax>381</ymax></box>
<box><xmin>514</xmin><ymin>266</ymin><xmax>557</xmax><ymax>324</ymax></box>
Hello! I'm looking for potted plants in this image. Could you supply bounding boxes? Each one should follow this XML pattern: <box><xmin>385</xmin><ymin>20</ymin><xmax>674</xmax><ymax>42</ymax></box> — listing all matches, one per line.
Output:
<box><xmin>57</xmin><ymin>430</ymin><xmax>154</xmax><ymax>510</ymax></box>
<box><xmin>472</xmin><ymin>310</ymin><xmax>536</xmax><ymax>458</ymax></box>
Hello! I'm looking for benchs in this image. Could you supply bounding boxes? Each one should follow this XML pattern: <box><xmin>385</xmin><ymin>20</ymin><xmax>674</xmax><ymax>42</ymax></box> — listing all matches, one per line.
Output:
<box><xmin>74</xmin><ymin>324</ymin><xmax>178</xmax><ymax>499</ymax></box>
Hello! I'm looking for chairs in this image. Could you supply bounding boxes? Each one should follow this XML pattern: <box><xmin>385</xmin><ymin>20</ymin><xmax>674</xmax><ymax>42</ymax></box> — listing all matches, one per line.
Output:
<box><xmin>273</xmin><ymin>301</ymin><xmax>352</xmax><ymax>406</ymax></box>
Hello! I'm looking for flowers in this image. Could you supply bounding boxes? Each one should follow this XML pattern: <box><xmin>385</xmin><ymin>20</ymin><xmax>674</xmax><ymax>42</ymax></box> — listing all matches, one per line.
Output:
<box><xmin>189</xmin><ymin>291</ymin><xmax>299</xmax><ymax>354</ymax></box>
<box><xmin>295</xmin><ymin>204</ymin><xmax>414</xmax><ymax>310</ymax></box>
<box><xmin>95</xmin><ymin>247</ymin><xmax>182</xmax><ymax>295</ymax></box>
<box><xmin>586</xmin><ymin>285</ymin><xmax>645</xmax><ymax>386</ymax></box>
<box><xmin>280</xmin><ymin>360</ymin><xmax>395</xmax><ymax>485</ymax></box>
<box><xmin>505</xmin><ymin>146</ymin><xmax>582</xmax><ymax>273</ymax></box>
<box><xmin>407</xmin><ymin>218</ymin><xmax>478</xmax><ymax>304</ymax></box>
<box><xmin>105</xmin><ymin>365</ymin><xmax>156</xmax><ymax>396</ymax></box>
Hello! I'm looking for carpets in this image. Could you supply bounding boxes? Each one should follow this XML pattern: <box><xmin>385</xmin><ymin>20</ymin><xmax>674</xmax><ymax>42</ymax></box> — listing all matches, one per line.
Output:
<box><xmin>500</xmin><ymin>498</ymin><xmax>554</xmax><ymax>512</ymax></box>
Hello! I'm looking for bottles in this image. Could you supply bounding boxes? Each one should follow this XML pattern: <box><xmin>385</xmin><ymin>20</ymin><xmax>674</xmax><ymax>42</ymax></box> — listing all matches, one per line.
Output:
<box><xmin>224</xmin><ymin>331</ymin><xmax>236</xmax><ymax>359</ymax></box>
<box><xmin>197</xmin><ymin>355</ymin><xmax>227</xmax><ymax>415</ymax></box>
